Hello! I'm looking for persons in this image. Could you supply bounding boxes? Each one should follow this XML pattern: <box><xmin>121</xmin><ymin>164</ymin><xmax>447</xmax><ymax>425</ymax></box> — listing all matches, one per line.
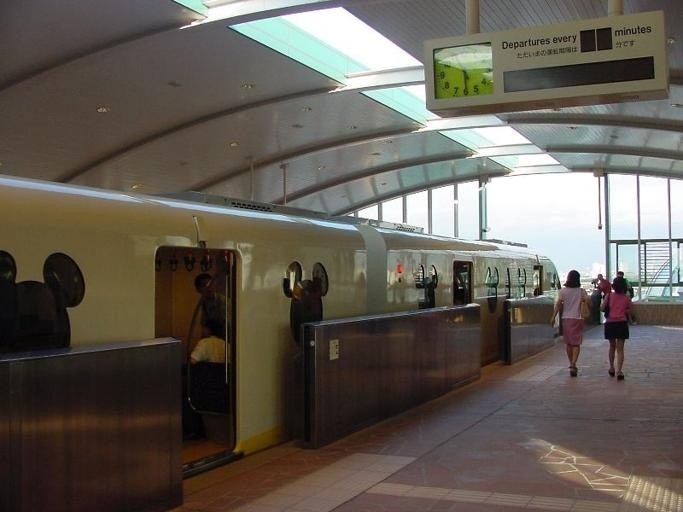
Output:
<box><xmin>193</xmin><ymin>272</ymin><xmax>231</xmax><ymax>344</ymax></box>
<box><xmin>599</xmin><ymin>277</ymin><xmax>637</xmax><ymax>380</ymax></box>
<box><xmin>183</xmin><ymin>316</ymin><xmax>231</xmax><ymax>365</ymax></box>
<box><xmin>615</xmin><ymin>272</ymin><xmax>639</xmax><ymax>326</ymax></box>
<box><xmin>546</xmin><ymin>270</ymin><xmax>591</xmax><ymax>378</ymax></box>
<box><xmin>591</xmin><ymin>274</ymin><xmax>612</xmax><ymax>300</ymax></box>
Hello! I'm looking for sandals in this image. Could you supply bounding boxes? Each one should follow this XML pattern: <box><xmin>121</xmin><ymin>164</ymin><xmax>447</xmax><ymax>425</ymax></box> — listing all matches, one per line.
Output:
<box><xmin>608</xmin><ymin>368</ymin><xmax>616</xmax><ymax>377</ymax></box>
<box><xmin>569</xmin><ymin>366</ymin><xmax>578</xmax><ymax>376</ymax></box>
<box><xmin>617</xmin><ymin>371</ymin><xmax>624</xmax><ymax>380</ymax></box>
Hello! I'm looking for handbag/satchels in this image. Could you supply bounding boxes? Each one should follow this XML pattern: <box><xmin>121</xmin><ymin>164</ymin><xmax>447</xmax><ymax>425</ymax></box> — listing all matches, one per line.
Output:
<box><xmin>604</xmin><ymin>304</ymin><xmax>609</xmax><ymax>318</ymax></box>
<box><xmin>580</xmin><ymin>301</ymin><xmax>590</xmax><ymax>318</ymax></box>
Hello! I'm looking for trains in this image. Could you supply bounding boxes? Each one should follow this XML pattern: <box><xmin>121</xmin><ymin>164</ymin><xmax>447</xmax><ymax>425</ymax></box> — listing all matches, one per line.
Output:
<box><xmin>0</xmin><ymin>172</ymin><xmax>563</xmax><ymax>480</ymax></box>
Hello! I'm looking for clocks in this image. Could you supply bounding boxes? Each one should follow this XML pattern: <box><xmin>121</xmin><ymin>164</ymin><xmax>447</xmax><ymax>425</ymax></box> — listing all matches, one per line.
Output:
<box><xmin>432</xmin><ymin>41</ymin><xmax>493</xmax><ymax>101</ymax></box>
